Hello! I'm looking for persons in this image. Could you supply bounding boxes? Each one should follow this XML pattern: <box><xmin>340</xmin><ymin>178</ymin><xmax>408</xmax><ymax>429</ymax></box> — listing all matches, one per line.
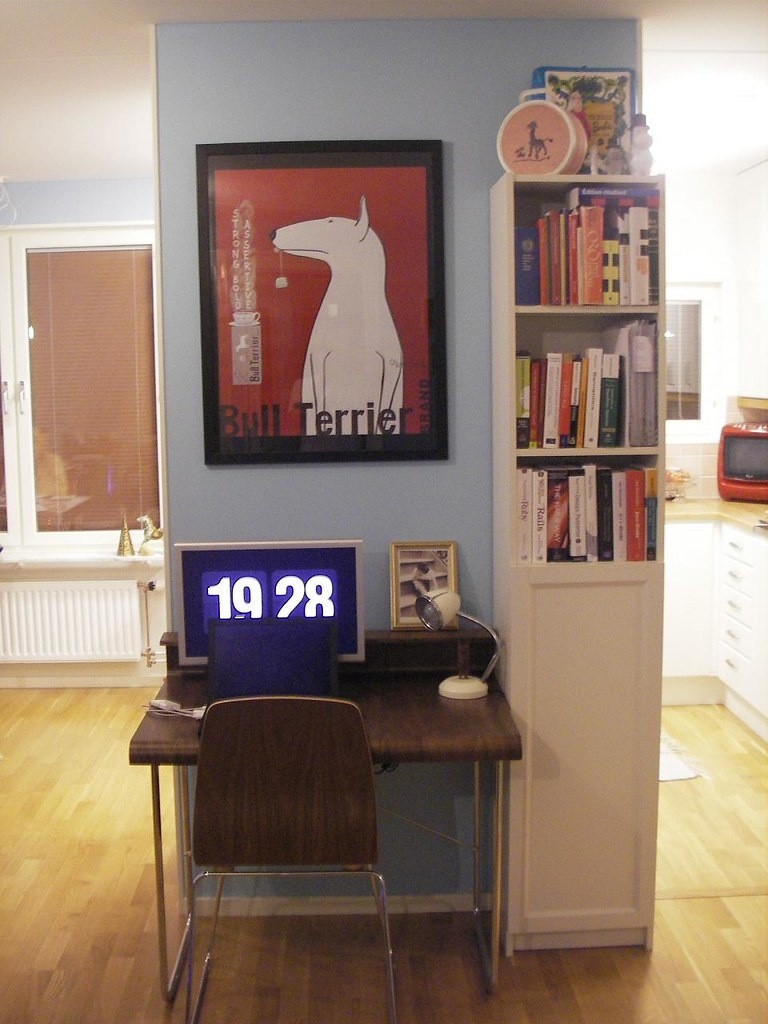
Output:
<box><xmin>413</xmin><ymin>563</ymin><xmax>437</xmax><ymax>596</ymax></box>
<box><xmin>2</xmin><ymin>425</ymin><xmax>68</xmax><ymax>498</ymax></box>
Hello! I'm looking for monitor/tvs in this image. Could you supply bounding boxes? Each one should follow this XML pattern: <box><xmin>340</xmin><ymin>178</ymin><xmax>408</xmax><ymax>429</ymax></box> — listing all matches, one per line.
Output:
<box><xmin>717</xmin><ymin>420</ymin><xmax>768</xmax><ymax>502</ymax></box>
<box><xmin>172</xmin><ymin>541</ymin><xmax>366</xmax><ymax>664</ymax></box>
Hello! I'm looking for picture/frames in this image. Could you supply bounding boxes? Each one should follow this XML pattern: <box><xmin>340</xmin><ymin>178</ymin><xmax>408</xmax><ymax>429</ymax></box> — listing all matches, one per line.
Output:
<box><xmin>390</xmin><ymin>540</ymin><xmax>459</xmax><ymax>632</ymax></box>
<box><xmin>195</xmin><ymin>139</ymin><xmax>448</xmax><ymax>465</ymax></box>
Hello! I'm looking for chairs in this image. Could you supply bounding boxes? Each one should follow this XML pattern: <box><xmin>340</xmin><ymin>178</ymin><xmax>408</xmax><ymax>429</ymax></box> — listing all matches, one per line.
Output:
<box><xmin>172</xmin><ymin>693</ymin><xmax>396</xmax><ymax>1024</ymax></box>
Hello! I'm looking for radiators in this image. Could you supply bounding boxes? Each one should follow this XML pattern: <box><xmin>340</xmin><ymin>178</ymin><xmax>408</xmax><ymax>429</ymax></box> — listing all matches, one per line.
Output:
<box><xmin>0</xmin><ymin>579</ymin><xmax>156</xmax><ymax>667</ymax></box>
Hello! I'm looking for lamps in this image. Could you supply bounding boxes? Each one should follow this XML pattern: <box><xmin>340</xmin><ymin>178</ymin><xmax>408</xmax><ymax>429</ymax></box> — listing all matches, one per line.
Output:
<box><xmin>414</xmin><ymin>589</ymin><xmax>500</xmax><ymax>699</ymax></box>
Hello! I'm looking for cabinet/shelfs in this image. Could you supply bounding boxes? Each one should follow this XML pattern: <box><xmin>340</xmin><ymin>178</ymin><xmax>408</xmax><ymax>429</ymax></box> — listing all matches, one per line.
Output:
<box><xmin>715</xmin><ymin>518</ymin><xmax>768</xmax><ymax>744</ymax></box>
<box><xmin>493</xmin><ymin>166</ymin><xmax>664</xmax><ymax>954</ymax></box>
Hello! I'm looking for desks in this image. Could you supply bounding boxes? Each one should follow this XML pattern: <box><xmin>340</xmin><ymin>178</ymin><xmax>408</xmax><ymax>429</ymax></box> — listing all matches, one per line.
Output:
<box><xmin>130</xmin><ymin>661</ymin><xmax>525</xmax><ymax>1000</ymax></box>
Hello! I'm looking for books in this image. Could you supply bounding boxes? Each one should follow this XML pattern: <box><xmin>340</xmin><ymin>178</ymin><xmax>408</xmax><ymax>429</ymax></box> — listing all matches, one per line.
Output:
<box><xmin>517</xmin><ymin>317</ymin><xmax>659</xmax><ymax>448</ymax></box>
<box><xmin>517</xmin><ymin>462</ymin><xmax>657</xmax><ymax>562</ymax></box>
<box><xmin>515</xmin><ymin>188</ymin><xmax>659</xmax><ymax>306</ymax></box>
